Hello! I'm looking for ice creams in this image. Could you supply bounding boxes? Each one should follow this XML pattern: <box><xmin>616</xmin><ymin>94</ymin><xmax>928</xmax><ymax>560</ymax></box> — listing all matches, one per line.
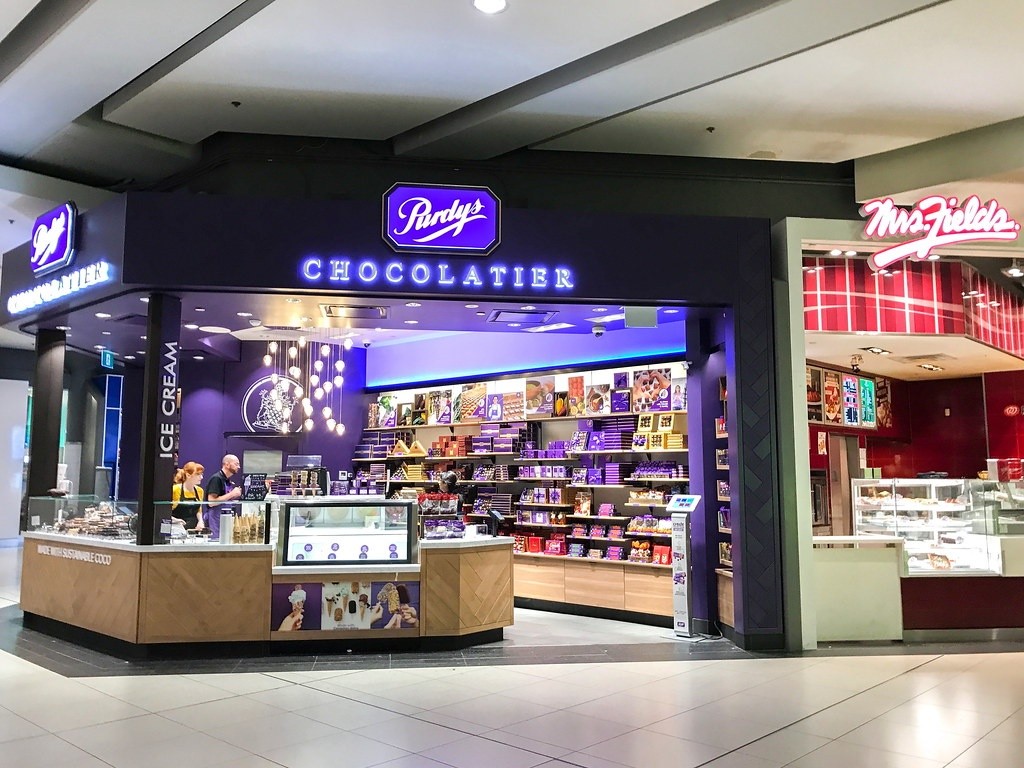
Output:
<box><xmin>288</xmin><ymin>582</ymin><xmax>410</xmax><ymax>624</ymax></box>
<box><xmin>244</xmin><ymin>475</ymin><xmax>251</xmax><ymax>494</ymax></box>
<box><xmin>289</xmin><ymin>470</ymin><xmax>321</xmax><ymax>497</ymax></box>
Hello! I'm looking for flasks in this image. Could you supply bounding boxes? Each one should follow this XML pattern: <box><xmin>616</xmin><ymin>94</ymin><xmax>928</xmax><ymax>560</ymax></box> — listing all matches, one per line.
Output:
<box><xmin>218</xmin><ymin>507</ymin><xmax>233</xmax><ymax>545</ymax></box>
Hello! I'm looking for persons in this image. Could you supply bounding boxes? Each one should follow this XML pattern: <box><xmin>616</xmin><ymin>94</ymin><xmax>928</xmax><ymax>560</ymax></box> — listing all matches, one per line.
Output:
<box><xmin>172</xmin><ymin>461</ymin><xmax>204</xmax><ymax>530</ymax></box>
<box><xmin>438</xmin><ymin>470</ymin><xmax>463</xmax><ymax>511</ymax></box>
<box><xmin>488</xmin><ymin>396</ymin><xmax>501</xmax><ymax>419</ymax></box>
<box><xmin>671</xmin><ymin>385</ymin><xmax>683</xmax><ymax>410</ymax></box>
<box><xmin>202</xmin><ymin>454</ymin><xmax>241</xmax><ymax>538</ymax></box>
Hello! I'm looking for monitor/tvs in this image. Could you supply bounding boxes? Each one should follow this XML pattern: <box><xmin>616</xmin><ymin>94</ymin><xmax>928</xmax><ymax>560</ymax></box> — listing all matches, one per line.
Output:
<box><xmin>284</xmin><ymin>501</ymin><xmax>413</xmax><ymax>563</ymax></box>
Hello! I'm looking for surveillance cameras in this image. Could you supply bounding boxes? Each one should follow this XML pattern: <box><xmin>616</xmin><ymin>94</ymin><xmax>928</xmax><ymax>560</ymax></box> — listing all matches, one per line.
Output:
<box><xmin>362</xmin><ymin>339</ymin><xmax>372</xmax><ymax>348</ymax></box>
<box><xmin>850</xmin><ymin>354</ymin><xmax>863</xmax><ymax>373</ymax></box>
<box><xmin>592</xmin><ymin>326</ymin><xmax>607</xmax><ymax>339</ymax></box>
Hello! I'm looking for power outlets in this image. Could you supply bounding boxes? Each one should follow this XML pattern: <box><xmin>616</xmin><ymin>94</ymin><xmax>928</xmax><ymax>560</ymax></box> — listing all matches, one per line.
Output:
<box><xmin>945</xmin><ymin>409</ymin><xmax>949</xmax><ymax>415</ymax></box>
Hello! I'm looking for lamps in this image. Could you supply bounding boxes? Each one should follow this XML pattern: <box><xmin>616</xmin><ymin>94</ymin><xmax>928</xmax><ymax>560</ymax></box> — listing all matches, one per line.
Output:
<box><xmin>263</xmin><ymin>328</ymin><xmax>353</xmax><ymax>436</ymax></box>
<box><xmin>1000</xmin><ymin>257</ymin><xmax>1024</xmax><ymax>279</ymax></box>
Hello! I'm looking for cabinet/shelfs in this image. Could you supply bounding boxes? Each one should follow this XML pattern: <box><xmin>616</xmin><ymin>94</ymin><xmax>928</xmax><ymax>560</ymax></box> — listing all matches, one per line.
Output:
<box><xmin>351</xmin><ymin>409</ymin><xmax>734</xmax><ymax>640</ymax></box>
<box><xmin>851</xmin><ymin>477</ymin><xmax>1023</xmax><ymax>577</ymax></box>
